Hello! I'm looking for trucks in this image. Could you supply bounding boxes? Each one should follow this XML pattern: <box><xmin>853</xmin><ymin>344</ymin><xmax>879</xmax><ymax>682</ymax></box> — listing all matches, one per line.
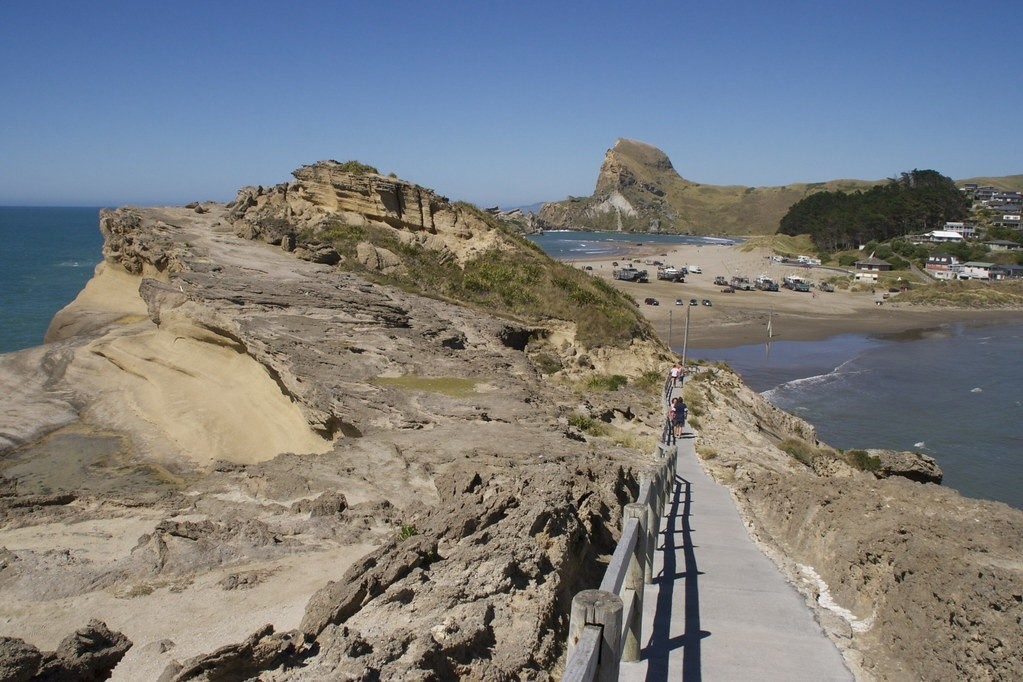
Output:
<box><xmin>615</xmin><ymin>263</ymin><xmax>648</xmax><ymax>282</ymax></box>
<box><xmin>714</xmin><ymin>276</ymin><xmax>834</xmax><ymax>293</ymax></box>
<box><xmin>657</xmin><ymin>266</ymin><xmax>685</xmax><ymax>283</ymax></box>
<box><xmin>689</xmin><ymin>265</ymin><xmax>701</xmax><ymax>274</ymax></box>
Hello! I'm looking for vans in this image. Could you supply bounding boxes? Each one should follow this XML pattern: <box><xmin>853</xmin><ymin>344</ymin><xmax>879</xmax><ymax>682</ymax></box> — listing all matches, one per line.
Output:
<box><xmin>883</xmin><ymin>293</ymin><xmax>890</xmax><ymax>299</ymax></box>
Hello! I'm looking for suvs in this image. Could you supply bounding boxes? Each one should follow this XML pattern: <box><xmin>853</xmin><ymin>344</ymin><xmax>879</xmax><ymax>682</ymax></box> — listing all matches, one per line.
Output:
<box><xmin>645</xmin><ymin>298</ymin><xmax>658</xmax><ymax>305</ymax></box>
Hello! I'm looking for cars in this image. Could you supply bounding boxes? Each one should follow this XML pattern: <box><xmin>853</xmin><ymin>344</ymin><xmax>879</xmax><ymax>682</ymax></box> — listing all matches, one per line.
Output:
<box><xmin>690</xmin><ymin>299</ymin><xmax>697</xmax><ymax>305</ymax></box>
<box><xmin>675</xmin><ymin>299</ymin><xmax>683</xmax><ymax>305</ymax></box>
<box><xmin>702</xmin><ymin>300</ymin><xmax>711</xmax><ymax>306</ymax></box>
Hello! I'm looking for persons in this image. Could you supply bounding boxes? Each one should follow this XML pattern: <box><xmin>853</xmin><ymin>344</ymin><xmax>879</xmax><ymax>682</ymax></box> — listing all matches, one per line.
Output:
<box><xmin>670</xmin><ymin>364</ymin><xmax>685</xmax><ymax>388</ymax></box>
<box><xmin>668</xmin><ymin>397</ymin><xmax>688</xmax><ymax>438</ymax></box>
